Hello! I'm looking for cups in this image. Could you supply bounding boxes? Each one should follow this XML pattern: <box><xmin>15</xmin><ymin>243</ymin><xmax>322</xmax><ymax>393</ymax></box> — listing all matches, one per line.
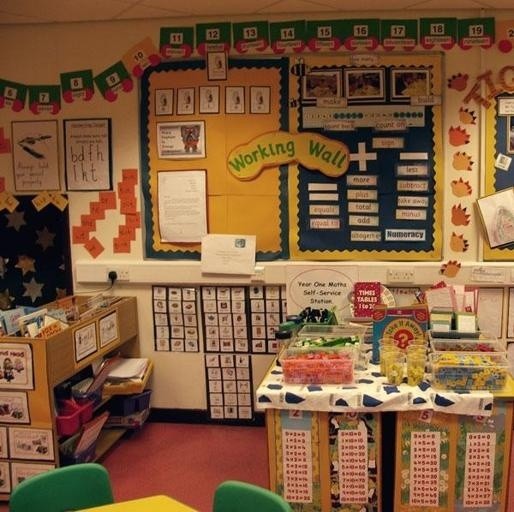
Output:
<box><xmin>378</xmin><ymin>338</ymin><xmax>429</xmax><ymax>387</ymax></box>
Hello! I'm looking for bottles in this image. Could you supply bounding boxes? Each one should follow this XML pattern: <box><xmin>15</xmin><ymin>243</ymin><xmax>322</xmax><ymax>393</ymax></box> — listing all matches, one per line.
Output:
<box><xmin>275</xmin><ymin>314</ymin><xmax>303</xmax><ymax>366</ymax></box>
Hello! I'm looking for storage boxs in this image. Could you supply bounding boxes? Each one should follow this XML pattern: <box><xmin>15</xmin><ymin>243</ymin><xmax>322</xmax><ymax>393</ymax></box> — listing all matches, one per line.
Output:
<box><xmin>276</xmin><ymin>319</ymin><xmax>512</xmax><ymax>393</ymax></box>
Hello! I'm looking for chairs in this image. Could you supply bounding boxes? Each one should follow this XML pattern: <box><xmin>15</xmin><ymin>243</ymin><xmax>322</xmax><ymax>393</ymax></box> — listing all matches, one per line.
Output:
<box><xmin>7</xmin><ymin>462</ymin><xmax>114</xmax><ymax>509</ymax></box>
<box><xmin>212</xmin><ymin>479</ymin><xmax>293</xmax><ymax>512</ymax></box>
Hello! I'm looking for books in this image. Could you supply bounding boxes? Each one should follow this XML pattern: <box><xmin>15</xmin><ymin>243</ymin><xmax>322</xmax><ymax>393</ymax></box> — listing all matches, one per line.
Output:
<box><xmin>0</xmin><ymin>292</ymin><xmax>125</xmax><ymax>339</ymax></box>
<box><xmin>71</xmin><ymin>355</ymin><xmax>150</xmax><ymax>457</ymax></box>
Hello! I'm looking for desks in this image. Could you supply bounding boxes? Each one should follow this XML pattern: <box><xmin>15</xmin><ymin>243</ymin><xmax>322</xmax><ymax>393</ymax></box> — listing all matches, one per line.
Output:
<box><xmin>257</xmin><ymin>351</ymin><xmax>512</xmax><ymax>512</ymax></box>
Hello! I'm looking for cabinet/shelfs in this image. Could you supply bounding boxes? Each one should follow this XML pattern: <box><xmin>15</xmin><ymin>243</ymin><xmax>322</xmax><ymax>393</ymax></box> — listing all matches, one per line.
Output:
<box><xmin>0</xmin><ymin>296</ymin><xmax>149</xmax><ymax>503</ymax></box>
